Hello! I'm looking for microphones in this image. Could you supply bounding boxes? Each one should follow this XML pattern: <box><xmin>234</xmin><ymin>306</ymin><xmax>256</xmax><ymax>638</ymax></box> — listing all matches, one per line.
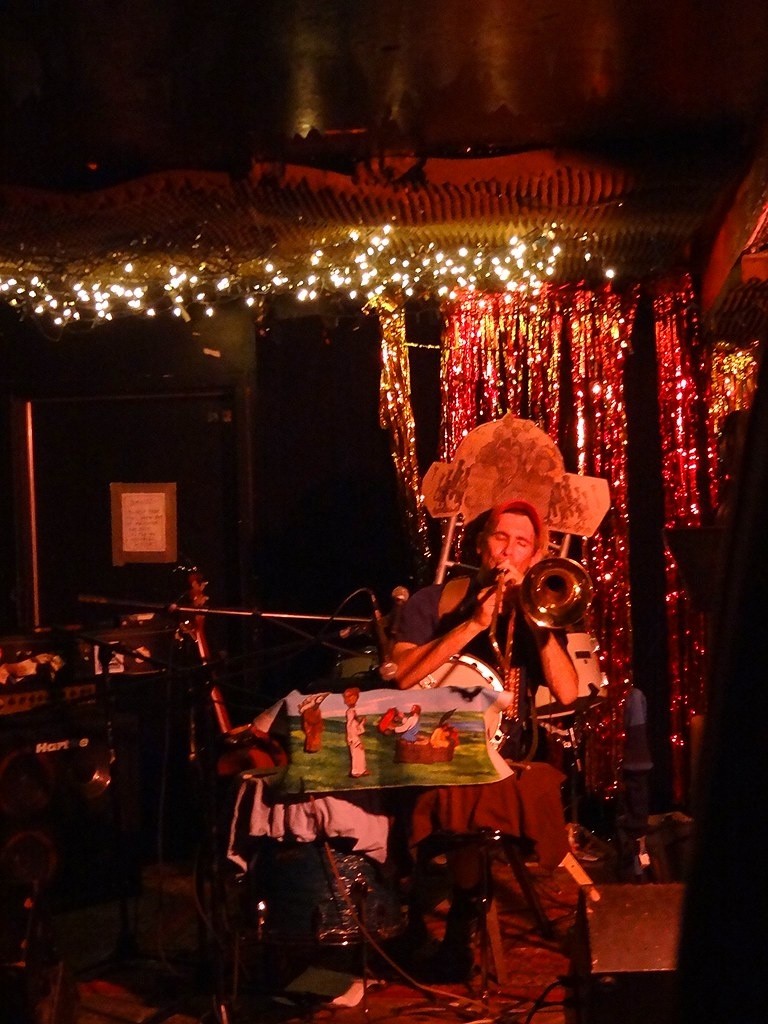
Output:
<box><xmin>373</xmin><ymin>612</ymin><xmax>399</xmax><ymax>681</ymax></box>
<box><xmin>388</xmin><ymin>587</ymin><xmax>409</xmax><ymax>644</ymax></box>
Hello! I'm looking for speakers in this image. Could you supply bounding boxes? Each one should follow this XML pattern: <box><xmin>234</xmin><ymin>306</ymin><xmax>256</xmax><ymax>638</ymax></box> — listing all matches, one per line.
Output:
<box><xmin>564</xmin><ymin>881</ymin><xmax>684</xmax><ymax>1024</ymax></box>
<box><xmin>12</xmin><ymin>384</ymin><xmax>242</xmax><ymax>632</ymax></box>
<box><xmin>0</xmin><ymin>710</ymin><xmax>143</xmax><ymax>922</ymax></box>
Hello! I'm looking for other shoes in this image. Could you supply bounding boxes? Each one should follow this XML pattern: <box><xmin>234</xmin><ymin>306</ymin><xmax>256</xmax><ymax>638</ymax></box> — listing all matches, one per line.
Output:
<box><xmin>394</xmin><ymin>921</ymin><xmax>429</xmax><ymax>962</ymax></box>
<box><xmin>433</xmin><ymin>939</ymin><xmax>467</xmax><ymax>981</ymax></box>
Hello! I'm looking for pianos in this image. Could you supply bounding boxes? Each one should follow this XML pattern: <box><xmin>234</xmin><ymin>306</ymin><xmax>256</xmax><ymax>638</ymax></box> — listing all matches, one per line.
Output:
<box><xmin>0</xmin><ymin>613</ymin><xmax>182</xmax><ymax>715</ymax></box>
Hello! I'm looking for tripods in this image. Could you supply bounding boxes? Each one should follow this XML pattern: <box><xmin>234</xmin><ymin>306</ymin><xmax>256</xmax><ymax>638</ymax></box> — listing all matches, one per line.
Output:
<box><xmin>44</xmin><ymin>620</ymin><xmax>278</xmax><ymax>979</ymax></box>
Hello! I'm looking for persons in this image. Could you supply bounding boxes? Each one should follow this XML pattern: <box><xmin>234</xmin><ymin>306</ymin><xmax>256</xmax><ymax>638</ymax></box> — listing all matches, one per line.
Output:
<box><xmin>381</xmin><ymin>499</ymin><xmax>579</xmax><ymax>981</ymax></box>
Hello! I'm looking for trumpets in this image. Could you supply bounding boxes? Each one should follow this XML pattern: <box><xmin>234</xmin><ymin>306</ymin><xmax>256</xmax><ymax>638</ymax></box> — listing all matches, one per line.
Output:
<box><xmin>489</xmin><ymin>557</ymin><xmax>595</xmax><ymax>673</ymax></box>
